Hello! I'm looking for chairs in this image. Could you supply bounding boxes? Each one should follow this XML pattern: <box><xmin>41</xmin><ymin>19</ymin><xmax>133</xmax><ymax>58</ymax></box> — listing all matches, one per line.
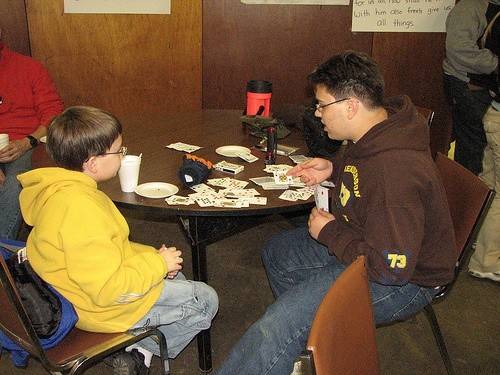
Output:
<box><xmin>300</xmin><ymin>256</ymin><xmax>380</xmax><ymax>375</ymax></box>
<box><xmin>0</xmin><ymin>250</ymin><xmax>171</xmax><ymax>375</ymax></box>
<box><xmin>375</xmin><ymin>152</ymin><xmax>497</xmax><ymax>375</ymax></box>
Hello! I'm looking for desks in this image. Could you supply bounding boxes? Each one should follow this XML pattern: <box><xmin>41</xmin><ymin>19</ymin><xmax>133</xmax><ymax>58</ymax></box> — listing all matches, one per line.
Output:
<box><xmin>31</xmin><ymin>108</ymin><xmax>331</xmax><ymax>375</ymax></box>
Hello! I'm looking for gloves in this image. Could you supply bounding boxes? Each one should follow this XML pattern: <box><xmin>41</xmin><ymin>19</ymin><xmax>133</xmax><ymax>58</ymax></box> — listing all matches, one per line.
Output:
<box><xmin>179</xmin><ymin>154</ymin><xmax>213</xmax><ymax>187</ymax></box>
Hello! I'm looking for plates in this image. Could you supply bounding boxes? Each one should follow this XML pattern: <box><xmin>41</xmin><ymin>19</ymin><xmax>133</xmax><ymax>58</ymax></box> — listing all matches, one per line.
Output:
<box><xmin>134</xmin><ymin>182</ymin><xmax>179</xmax><ymax>198</ymax></box>
<box><xmin>215</xmin><ymin>146</ymin><xmax>251</xmax><ymax>157</ymax></box>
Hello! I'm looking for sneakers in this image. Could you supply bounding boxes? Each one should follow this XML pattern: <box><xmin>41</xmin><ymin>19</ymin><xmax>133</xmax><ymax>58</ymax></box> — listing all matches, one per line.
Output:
<box><xmin>468</xmin><ymin>268</ymin><xmax>500</xmax><ymax>282</ymax></box>
<box><xmin>103</xmin><ymin>348</ymin><xmax>150</xmax><ymax>375</ymax></box>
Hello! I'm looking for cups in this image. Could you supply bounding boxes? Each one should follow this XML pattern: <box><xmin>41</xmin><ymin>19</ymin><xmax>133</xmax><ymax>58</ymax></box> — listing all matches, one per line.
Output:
<box><xmin>0</xmin><ymin>134</ymin><xmax>9</xmax><ymax>162</ymax></box>
<box><xmin>118</xmin><ymin>155</ymin><xmax>141</xmax><ymax>192</ymax></box>
<box><xmin>246</xmin><ymin>79</ymin><xmax>272</xmax><ymax>116</ymax></box>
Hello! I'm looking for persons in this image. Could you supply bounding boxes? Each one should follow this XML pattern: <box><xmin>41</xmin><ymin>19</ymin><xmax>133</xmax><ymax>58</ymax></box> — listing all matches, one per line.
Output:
<box><xmin>0</xmin><ymin>41</ymin><xmax>63</xmax><ymax>259</ymax></box>
<box><xmin>219</xmin><ymin>51</ymin><xmax>458</xmax><ymax>375</ymax></box>
<box><xmin>17</xmin><ymin>106</ymin><xmax>219</xmax><ymax>375</ymax></box>
<box><xmin>441</xmin><ymin>1</ymin><xmax>500</xmax><ymax>283</ymax></box>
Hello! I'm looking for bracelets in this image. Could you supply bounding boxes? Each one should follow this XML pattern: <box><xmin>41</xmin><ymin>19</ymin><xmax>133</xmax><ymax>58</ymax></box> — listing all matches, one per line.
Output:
<box><xmin>27</xmin><ymin>135</ymin><xmax>38</xmax><ymax>148</ymax></box>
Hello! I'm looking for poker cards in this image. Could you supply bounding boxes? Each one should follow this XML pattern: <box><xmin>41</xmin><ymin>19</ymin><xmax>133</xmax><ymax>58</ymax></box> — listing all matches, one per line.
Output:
<box><xmin>314</xmin><ymin>190</ymin><xmax>328</xmax><ymax>212</ymax></box>
<box><xmin>249</xmin><ymin>155</ymin><xmax>335</xmax><ymax>202</ymax></box>
<box><xmin>235</xmin><ymin>150</ymin><xmax>259</xmax><ymax>163</ymax></box>
<box><xmin>166</xmin><ymin>142</ymin><xmax>201</xmax><ymax>154</ymax></box>
<box><xmin>165</xmin><ymin>177</ymin><xmax>267</xmax><ymax>209</ymax></box>
<box><xmin>273</xmin><ymin>171</ymin><xmax>294</xmax><ymax>185</ymax></box>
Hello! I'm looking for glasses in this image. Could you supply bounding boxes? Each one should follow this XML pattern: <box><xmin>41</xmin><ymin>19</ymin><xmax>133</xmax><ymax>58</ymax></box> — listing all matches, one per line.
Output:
<box><xmin>316</xmin><ymin>98</ymin><xmax>351</xmax><ymax>112</ymax></box>
<box><xmin>85</xmin><ymin>146</ymin><xmax>127</xmax><ymax>162</ymax></box>
